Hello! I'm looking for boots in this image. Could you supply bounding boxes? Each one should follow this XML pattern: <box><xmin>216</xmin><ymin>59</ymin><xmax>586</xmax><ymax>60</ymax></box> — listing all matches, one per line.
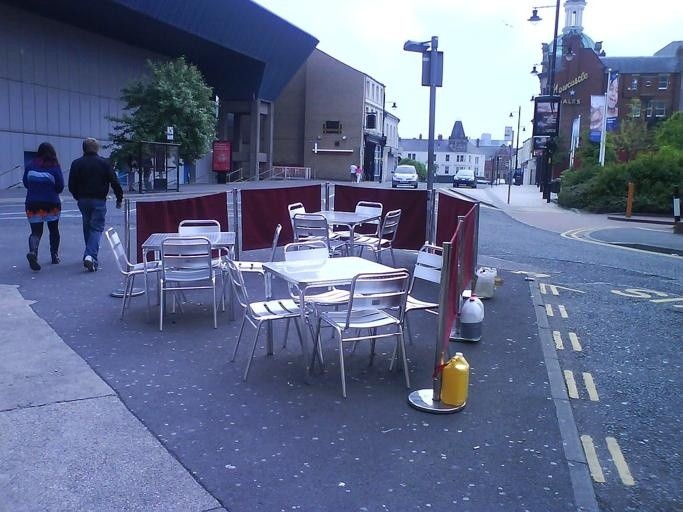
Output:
<box><xmin>26</xmin><ymin>234</ymin><xmax>41</xmax><ymax>271</ymax></box>
<box><xmin>49</xmin><ymin>233</ymin><xmax>61</xmax><ymax>265</ymax></box>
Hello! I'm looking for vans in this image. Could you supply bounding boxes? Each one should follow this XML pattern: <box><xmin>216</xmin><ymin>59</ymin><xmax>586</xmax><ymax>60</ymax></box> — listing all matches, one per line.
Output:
<box><xmin>504</xmin><ymin>168</ymin><xmax>523</xmax><ymax>186</ymax></box>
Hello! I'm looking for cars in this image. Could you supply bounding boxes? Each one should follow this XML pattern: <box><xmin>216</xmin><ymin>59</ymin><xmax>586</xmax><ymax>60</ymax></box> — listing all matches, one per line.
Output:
<box><xmin>452</xmin><ymin>169</ymin><xmax>477</xmax><ymax>188</ymax></box>
<box><xmin>391</xmin><ymin>165</ymin><xmax>419</xmax><ymax>188</ymax></box>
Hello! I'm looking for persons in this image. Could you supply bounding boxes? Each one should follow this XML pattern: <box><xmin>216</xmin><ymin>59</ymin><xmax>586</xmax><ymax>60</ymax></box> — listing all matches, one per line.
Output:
<box><xmin>349</xmin><ymin>164</ymin><xmax>357</xmax><ymax>183</ymax></box>
<box><xmin>355</xmin><ymin>165</ymin><xmax>363</xmax><ymax>183</ymax></box>
<box><xmin>22</xmin><ymin>142</ymin><xmax>65</xmax><ymax>271</ymax></box>
<box><xmin>68</xmin><ymin>138</ymin><xmax>123</xmax><ymax>272</ymax></box>
<box><xmin>607</xmin><ymin>79</ymin><xmax>618</xmax><ymax>118</ymax></box>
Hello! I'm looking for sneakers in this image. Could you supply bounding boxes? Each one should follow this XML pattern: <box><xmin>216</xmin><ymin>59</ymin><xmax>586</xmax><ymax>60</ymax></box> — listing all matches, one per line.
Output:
<box><xmin>84</xmin><ymin>255</ymin><xmax>99</xmax><ymax>272</ymax></box>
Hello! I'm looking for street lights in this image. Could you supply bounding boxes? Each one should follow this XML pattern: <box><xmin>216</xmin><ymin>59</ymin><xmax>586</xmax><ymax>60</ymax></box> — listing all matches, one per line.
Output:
<box><xmin>508</xmin><ymin>104</ymin><xmax>522</xmax><ymax>173</ymax></box>
<box><xmin>497</xmin><ymin>156</ymin><xmax>502</xmax><ymax>184</ymax></box>
<box><xmin>506</xmin><ymin>140</ymin><xmax>512</xmax><ymax>204</ymax></box>
<box><xmin>402</xmin><ymin>35</ymin><xmax>438</xmax><ymax>254</ymax></box>
<box><xmin>524</xmin><ymin>0</ymin><xmax>562</xmax><ymax>203</ymax></box>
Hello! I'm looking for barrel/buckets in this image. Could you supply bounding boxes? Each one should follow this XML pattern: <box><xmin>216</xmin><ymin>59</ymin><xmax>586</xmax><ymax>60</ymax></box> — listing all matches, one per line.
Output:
<box><xmin>440</xmin><ymin>352</ymin><xmax>469</xmax><ymax>406</ymax></box>
<box><xmin>461</xmin><ymin>294</ymin><xmax>484</xmax><ymax>338</ymax></box>
<box><xmin>475</xmin><ymin>267</ymin><xmax>496</xmax><ymax>297</ymax></box>
